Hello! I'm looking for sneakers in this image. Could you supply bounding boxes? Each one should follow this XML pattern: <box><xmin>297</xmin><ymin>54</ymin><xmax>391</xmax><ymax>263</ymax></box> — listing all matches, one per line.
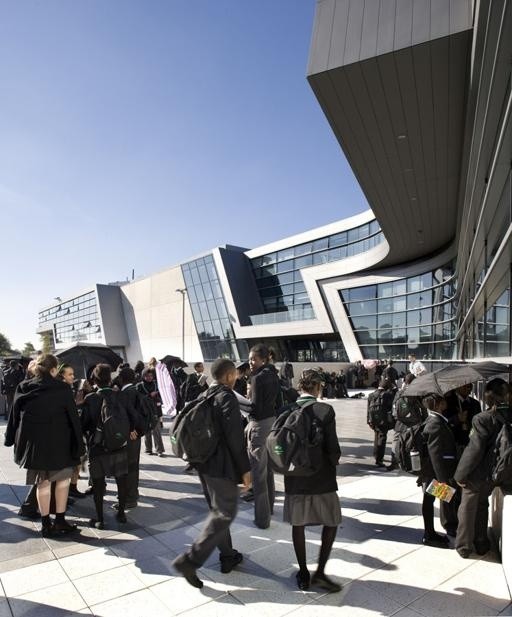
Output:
<box><xmin>156</xmin><ymin>451</ymin><xmax>163</xmax><ymax>457</ymax></box>
<box><xmin>145</xmin><ymin>450</ymin><xmax>153</xmax><ymax>455</ymax></box>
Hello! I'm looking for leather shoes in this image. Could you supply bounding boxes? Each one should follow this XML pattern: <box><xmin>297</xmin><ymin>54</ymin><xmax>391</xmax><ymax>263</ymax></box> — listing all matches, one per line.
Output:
<box><xmin>89</xmin><ymin>518</ymin><xmax>104</xmax><ymax>529</ymax></box>
<box><xmin>41</xmin><ymin>518</ymin><xmax>81</xmax><ymax>542</ymax></box>
<box><xmin>239</xmin><ymin>488</ymin><xmax>256</xmax><ymax>502</ymax></box>
<box><xmin>422</xmin><ymin>529</ymin><xmax>492</xmax><ymax>559</ymax></box>
<box><xmin>173</xmin><ymin>556</ymin><xmax>203</xmax><ymax>590</ymax></box>
<box><xmin>115</xmin><ymin>517</ymin><xmax>127</xmax><ymax>532</ymax></box>
<box><xmin>310</xmin><ymin>573</ymin><xmax>341</xmax><ymax>594</ymax></box>
<box><xmin>17</xmin><ymin>483</ymin><xmax>138</xmax><ymax>518</ymax></box>
<box><xmin>376</xmin><ymin>463</ymin><xmax>400</xmax><ymax>473</ymax></box>
<box><xmin>220</xmin><ymin>551</ymin><xmax>244</xmax><ymax>574</ymax></box>
<box><xmin>296</xmin><ymin>570</ymin><xmax>311</xmax><ymax>590</ymax></box>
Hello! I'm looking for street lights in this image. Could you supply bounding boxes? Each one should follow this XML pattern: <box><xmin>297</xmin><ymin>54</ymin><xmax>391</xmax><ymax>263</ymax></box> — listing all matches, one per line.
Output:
<box><xmin>175</xmin><ymin>289</ymin><xmax>185</xmax><ymax>362</ymax></box>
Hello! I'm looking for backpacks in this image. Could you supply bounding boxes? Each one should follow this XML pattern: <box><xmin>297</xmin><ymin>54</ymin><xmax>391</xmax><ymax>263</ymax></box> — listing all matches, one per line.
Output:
<box><xmin>265</xmin><ymin>400</ymin><xmax>327</xmax><ymax>478</ymax></box>
<box><xmin>366</xmin><ymin>389</ymin><xmax>391</xmax><ymax>433</ymax></box>
<box><xmin>391</xmin><ymin>415</ymin><xmax>434</xmax><ymax>476</ymax></box>
<box><xmin>169</xmin><ymin>385</ymin><xmax>237</xmax><ymax>465</ymax></box>
<box><xmin>395</xmin><ymin>396</ymin><xmax>421</xmax><ymax>427</ymax></box>
<box><xmin>486</xmin><ymin>408</ymin><xmax>512</xmax><ymax>486</ymax></box>
<box><xmin>88</xmin><ymin>389</ymin><xmax>131</xmax><ymax>453</ymax></box>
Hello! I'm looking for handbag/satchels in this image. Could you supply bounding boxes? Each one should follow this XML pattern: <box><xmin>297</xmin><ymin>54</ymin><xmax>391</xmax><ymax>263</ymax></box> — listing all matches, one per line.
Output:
<box><xmin>179</xmin><ymin>381</ymin><xmax>210</xmax><ymax>402</ymax></box>
